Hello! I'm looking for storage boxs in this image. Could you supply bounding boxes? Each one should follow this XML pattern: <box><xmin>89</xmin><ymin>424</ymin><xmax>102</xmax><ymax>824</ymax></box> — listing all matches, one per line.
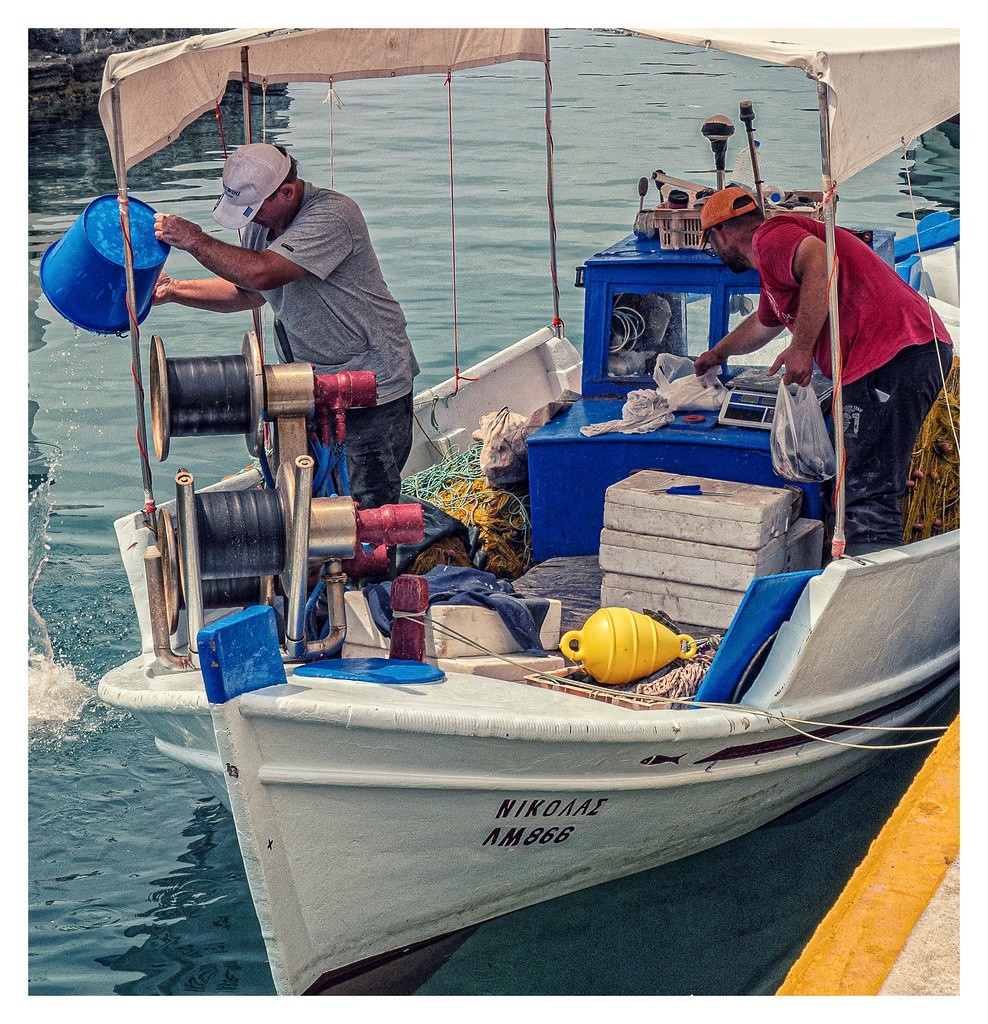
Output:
<box><xmin>654</xmin><ymin>187</ymin><xmax>838</xmax><ymax>248</ymax></box>
<box><xmin>596</xmin><ymin>468</ymin><xmax>827</xmax><ymax>635</ymax></box>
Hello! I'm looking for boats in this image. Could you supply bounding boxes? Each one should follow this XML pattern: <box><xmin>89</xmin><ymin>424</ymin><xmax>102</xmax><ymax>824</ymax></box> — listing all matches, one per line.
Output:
<box><xmin>82</xmin><ymin>32</ymin><xmax>959</xmax><ymax>999</ymax></box>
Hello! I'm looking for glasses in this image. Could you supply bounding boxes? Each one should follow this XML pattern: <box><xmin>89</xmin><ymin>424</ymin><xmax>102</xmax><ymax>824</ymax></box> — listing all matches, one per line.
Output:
<box><xmin>701</xmin><ymin>227</ymin><xmax>724</xmax><ymax>257</ymax></box>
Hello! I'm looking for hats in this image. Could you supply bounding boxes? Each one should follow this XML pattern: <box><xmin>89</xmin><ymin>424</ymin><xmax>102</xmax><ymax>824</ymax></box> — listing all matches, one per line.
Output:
<box><xmin>697</xmin><ymin>187</ymin><xmax>758</xmax><ymax>253</ymax></box>
<box><xmin>212</xmin><ymin>143</ymin><xmax>291</xmax><ymax>229</ymax></box>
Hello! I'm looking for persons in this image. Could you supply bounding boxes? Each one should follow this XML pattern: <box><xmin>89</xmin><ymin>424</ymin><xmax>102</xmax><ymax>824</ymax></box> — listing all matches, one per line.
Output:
<box><xmin>129</xmin><ymin>141</ymin><xmax>422</xmax><ymax>641</ymax></box>
<box><xmin>686</xmin><ymin>186</ymin><xmax>954</xmax><ymax>556</ymax></box>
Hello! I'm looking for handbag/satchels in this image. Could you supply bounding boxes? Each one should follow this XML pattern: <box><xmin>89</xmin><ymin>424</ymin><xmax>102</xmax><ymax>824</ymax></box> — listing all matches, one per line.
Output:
<box><xmin>654</xmin><ymin>350</ymin><xmax>728</xmax><ymax>411</ymax></box>
<box><xmin>771</xmin><ymin>374</ymin><xmax>837</xmax><ymax>484</ymax></box>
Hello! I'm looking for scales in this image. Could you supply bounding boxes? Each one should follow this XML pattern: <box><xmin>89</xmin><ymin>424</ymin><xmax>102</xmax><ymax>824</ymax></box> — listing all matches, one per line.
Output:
<box><xmin>717</xmin><ymin>367</ymin><xmax>835</xmax><ymax>432</ymax></box>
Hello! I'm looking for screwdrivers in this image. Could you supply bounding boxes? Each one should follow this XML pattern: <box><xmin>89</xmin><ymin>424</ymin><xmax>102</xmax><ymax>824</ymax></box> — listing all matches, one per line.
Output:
<box><xmin>649</xmin><ymin>485</ymin><xmax>702</xmax><ymax>492</ymax></box>
<box><xmin>666</xmin><ymin>489</ymin><xmax>734</xmax><ymax>497</ymax></box>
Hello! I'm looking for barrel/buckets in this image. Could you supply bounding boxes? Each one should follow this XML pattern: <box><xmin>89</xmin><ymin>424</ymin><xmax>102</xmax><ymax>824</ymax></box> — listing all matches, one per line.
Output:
<box><xmin>39</xmin><ymin>194</ymin><xmax>173</xmax><ymax>339</ymax></box>
